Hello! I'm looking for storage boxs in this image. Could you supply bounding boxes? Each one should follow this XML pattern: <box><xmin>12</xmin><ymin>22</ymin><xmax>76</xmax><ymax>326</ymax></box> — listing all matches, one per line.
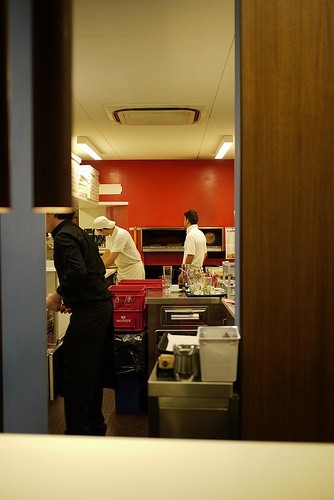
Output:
<box><xmin>198</xmin><ymin>326</ymin><xmax>241</xmax><ymax>382</ymax></box>
<box><xmin>108</xmin><ymin>278</ymin><xmax>162</xmax><ymax>330</ymax></box>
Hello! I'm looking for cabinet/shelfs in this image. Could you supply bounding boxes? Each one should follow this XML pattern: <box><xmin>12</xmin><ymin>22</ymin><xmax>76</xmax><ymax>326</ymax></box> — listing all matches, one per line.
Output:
<box><xmin>145</xmin><ymin>283</ymin><xmax>236</xmax><ymax>370</ymax></box>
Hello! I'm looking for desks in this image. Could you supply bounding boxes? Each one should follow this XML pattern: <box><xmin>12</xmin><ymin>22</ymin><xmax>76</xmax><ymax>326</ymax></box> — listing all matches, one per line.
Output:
<box><xmin>0</xmin><ymin>433</ymin><xmax>334</xmax><ymax>500</ymax></box>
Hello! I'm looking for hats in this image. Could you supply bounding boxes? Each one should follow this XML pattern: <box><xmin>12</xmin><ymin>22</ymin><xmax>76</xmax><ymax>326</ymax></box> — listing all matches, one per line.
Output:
<box><xmin>94</xmin><ymin>216</ymin><xmax>115</xmax><ymax>229</ymax></box>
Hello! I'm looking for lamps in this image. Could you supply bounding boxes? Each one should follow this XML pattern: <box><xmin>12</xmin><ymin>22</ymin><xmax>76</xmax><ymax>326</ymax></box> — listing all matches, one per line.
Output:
<box><xmin>77</xmin><ymin>137</ymin><xmax>102</xmax><ymax>160</ymax></box>
<box><xmin>215</xmin><ymin>135</ymin><xmax>233</xmax><ymax>159</ymax></box>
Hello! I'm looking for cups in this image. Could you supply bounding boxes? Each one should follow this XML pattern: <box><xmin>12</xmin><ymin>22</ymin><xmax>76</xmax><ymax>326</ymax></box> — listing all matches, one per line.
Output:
<box><xmin>184</xmin><ymin>268</ymin><xmax>212</xmax><ymax>295</ymax></box>
<box><xmin>163</xmin><ymin>265</ymin><xmax>172</xmax><ymax>285</ymax></box>
<box><xmin>161</xmin><ymin>275</ymin><xmax>171</xmax><ymax>296</ymax></box>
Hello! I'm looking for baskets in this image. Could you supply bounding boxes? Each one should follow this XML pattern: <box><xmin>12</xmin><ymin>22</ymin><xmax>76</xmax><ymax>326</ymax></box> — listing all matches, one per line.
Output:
<box><xmin>108</xmin><ymin>285</ymin><xmax>147</xmax><ymax>331</ymax></box>
<box><xmin>118</xmin><ymin>279</ymin><xmax>163</xmax><ymax>291</ymax></box>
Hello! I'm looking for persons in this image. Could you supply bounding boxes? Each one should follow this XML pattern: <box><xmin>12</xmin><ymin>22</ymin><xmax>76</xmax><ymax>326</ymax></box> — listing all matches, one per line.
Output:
<box><xmin>93</xmin><ymin>216</ymin><xmax>145</xmax><ymax>285</ymax></box>
<box><xmin>182</xmin><ymin>209</ymin><xmax>208</xmax><ymax>267</ymax></box>
<box><xmin>47</xmin><ymin>213</ymin><xmax>116</xmax><ymax>436</ymax></box>
